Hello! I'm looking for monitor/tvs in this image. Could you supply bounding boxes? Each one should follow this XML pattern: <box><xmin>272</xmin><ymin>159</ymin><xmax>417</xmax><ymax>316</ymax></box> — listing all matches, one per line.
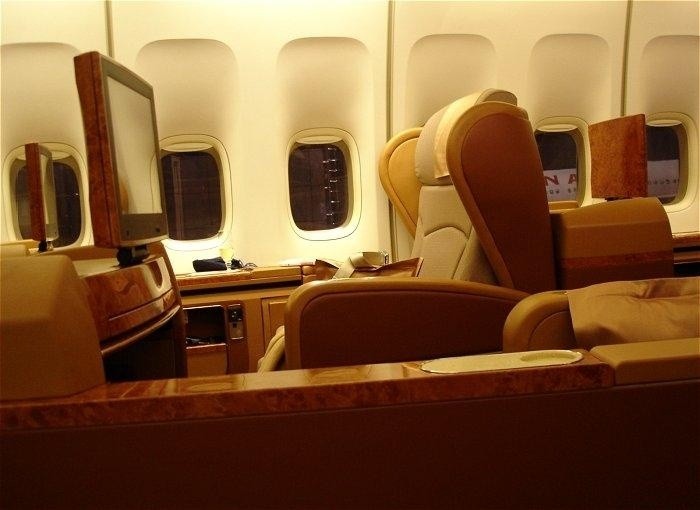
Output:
<box><xmin>72</xmin><ymin>50</ymin><xmax>170</xmax><ymax>265</ymax></box>
<box><xmin>24</xmin><ymin>142</ymin><xmax>60</xmax><ymax>251</ymax></box>
<box><xmin>587</xmin><ymin>114</ymin><xmax>648</xmax><ymax>201</ymax></box>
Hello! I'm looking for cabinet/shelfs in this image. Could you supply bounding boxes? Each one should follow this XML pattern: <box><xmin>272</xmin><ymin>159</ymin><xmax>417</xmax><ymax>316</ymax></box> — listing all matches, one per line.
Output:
<box><xmin>180</xmin><ymin>283</ymin><xmax>314</xmax><ymax>379</ymax></box>
